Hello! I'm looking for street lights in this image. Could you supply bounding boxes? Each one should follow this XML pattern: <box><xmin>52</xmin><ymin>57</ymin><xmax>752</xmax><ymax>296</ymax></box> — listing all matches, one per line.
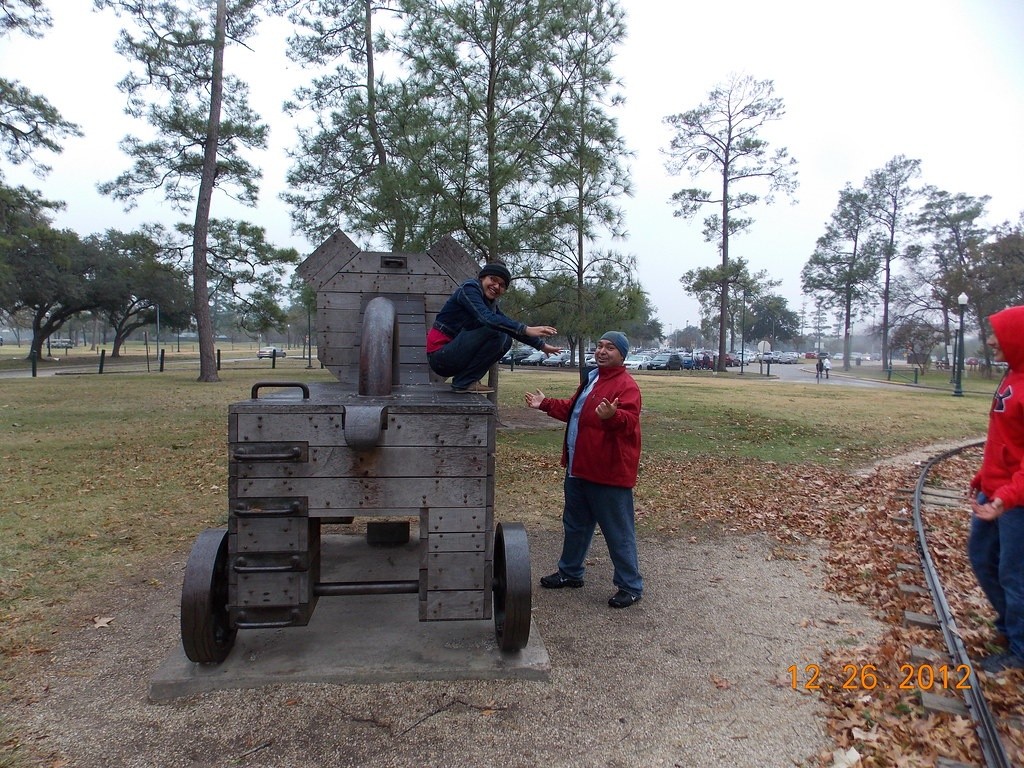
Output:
<box><xmin>954</xmin><ymin>291</ymin><xmax>970</xmax><ymax>396</ymax></box>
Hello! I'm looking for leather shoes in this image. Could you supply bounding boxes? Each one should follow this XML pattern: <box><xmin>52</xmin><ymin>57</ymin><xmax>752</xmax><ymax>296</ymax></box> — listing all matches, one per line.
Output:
<box><xmin>454</xmin><ymin>381</ymin><xmax>496</xmax><ymax>394</ymax></box>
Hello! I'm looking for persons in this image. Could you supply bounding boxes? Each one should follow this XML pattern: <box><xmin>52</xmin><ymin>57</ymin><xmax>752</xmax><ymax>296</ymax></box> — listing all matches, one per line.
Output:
<box><xmin>962</xmin><ymin>306</ymin><xmax>1024</xmax><ymax>674</ymax></box>
<box><xmin>936</xmin><ymin>359</ymin><xmax>943</xmax><ymax>371</ymax></box>
<box><xmin>694</xmin><ymin>352</ymin><xmax>700</xmax><ymax>370</ymax></box>
<box><xmin>523</xmin><ymin>330</ymin><xmax>646</xmax><ymax>609</ymax></box>
<box><xmin>424</xmin><ymin>262</ymin><xmax>563</xmax><ymax>396</ymax></box>
<box><xmin>816</xmin><ymin>358</ymin><xmax>823</xmax><ymax>378</ymax></box>
<box><xmin>701</xmin><ymin>351</ymin><xmax>707</xmax><ymax>370</ymax></box>
<box><xmin>705</xmin><ymin>355</ymin><xmax>710</xmax><ymax>370</ymax></box>
<box><xmin>823</xmin><ymin>358</ymin><xmax>832</xmax><ymax>379</ymax></box>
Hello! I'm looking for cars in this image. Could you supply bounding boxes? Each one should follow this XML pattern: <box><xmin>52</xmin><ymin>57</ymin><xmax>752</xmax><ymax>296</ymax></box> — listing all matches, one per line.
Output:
<box><xmin>502</xmin><ymin>344</ymin><xmax>883</xmax><ymax>373</ymax></box>
<box><xmin>935</xmin><ymin>356</ymin><xmax>1007</xmax><ymax>369</ymax></box>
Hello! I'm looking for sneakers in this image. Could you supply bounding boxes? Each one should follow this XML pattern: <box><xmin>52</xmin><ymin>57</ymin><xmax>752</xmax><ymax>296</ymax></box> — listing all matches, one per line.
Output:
<box><xmin>540</xmin><ymin>572</ymin><xmax>584</xmax><ymax>588</ymax></box>
<box><xmin>608</xmin><ymin>590</ymin><xmax>640</xmax><ymax>608</ymax></box>
<box><xmin>976</xmin><ymin>652</ymin><xmax>1024</xmax><ymax>678</ymax></box>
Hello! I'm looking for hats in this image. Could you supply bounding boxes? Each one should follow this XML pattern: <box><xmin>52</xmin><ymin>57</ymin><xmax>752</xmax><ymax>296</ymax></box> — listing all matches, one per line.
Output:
<box><xmin>600</xmin><ymin>331</ymin><xmax>629</xmax><ymax>360</ymax></box>
<box><xmin>479</xmin><ymin>259</ymin><xmax>509</xmax><ymax>289</ymax></box>
